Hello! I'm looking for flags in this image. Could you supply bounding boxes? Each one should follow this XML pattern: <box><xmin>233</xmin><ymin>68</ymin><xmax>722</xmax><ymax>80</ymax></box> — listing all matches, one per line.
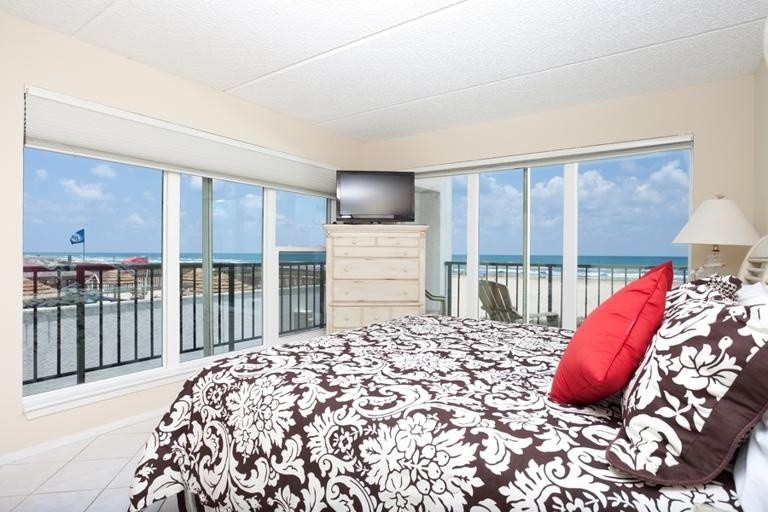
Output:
<box><xmin>69</xmin><ymin>229</ymin><xmax>84</xmax><ymax>244</ymax></box>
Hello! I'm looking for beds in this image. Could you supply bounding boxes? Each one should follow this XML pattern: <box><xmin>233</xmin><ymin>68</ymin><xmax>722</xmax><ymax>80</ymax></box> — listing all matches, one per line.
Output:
<box><xmin>121</xmin><ymin>272</ymin><xmax>768</xmax><ymax>512</ymax></box>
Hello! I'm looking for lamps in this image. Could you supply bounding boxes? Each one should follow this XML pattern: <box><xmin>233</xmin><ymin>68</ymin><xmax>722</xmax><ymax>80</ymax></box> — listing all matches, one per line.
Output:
<box><xmin>673</xmin><ymin>195</ymin><xmax>759</xmax><ymax>278</ymax></box>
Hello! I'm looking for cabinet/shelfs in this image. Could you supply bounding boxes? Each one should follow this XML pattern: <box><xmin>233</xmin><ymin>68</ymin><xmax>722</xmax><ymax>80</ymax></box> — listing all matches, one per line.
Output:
<box><xmin>323</xmin><ymin>221</ymin><xmax>429</xmax><ymax>334</ymax></box>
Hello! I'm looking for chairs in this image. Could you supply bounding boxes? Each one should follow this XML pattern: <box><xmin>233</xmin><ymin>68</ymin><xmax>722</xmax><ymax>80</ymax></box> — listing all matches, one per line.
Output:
<box><xmin>478</xmin><ymin>278</ymin><xmax>558</xmax><ymax>325</ymax></box>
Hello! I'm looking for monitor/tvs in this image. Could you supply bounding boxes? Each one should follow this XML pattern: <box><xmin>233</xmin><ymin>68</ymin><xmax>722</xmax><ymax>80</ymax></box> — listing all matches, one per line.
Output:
<box><xmin>335</xmin><ymin>170</ymin><xmax>415</xmax><ymax>224</ymax></box>
